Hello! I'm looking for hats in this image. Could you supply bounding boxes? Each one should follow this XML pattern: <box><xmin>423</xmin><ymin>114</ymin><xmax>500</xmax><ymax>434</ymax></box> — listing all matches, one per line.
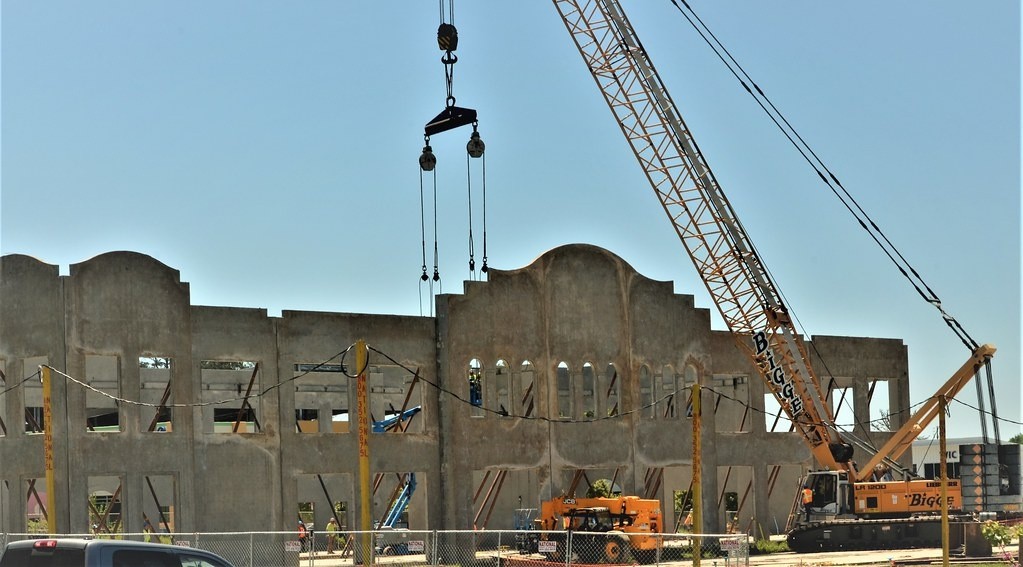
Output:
<box><xmin>144</xmin><ymin>521</ymin><xmax>148</xmax><ymax>527</ymax></box>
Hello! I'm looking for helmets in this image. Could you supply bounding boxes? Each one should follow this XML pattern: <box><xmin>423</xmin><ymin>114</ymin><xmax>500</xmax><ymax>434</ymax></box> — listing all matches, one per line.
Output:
<box><xmin>298</xmin><ymin>521</ymin><xmax>302</xmax><ymax>525</ymax></box>
<box><xmin>330</xmin><ymin>518</ymin><xmax>335</xmax><ymax>521</ymax></box>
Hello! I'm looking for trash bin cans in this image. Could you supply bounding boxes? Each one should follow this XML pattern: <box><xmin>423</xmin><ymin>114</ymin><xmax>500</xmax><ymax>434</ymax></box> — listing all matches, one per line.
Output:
<box><xmin>966</xmin><ymin>522</ymin><xmax>994</xmax><ymax>557</ymax></box>
<box><xmin>947</xmin><ymin>521</ymin><xmax>963</xmax><ymax>553</ymax></box>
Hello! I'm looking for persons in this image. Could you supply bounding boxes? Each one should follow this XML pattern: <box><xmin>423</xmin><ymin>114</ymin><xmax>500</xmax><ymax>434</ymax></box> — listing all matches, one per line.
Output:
<box><xmin>299</xmin><ymin>521</ymin><xmax>305</xmax><ymax>553</ymax></box>
<box><xmin>142</xmin><ymin>522</ymin><xmax>150</xmax><ymax>542</ymax></box>
<box><xmin>326</xmin><ymin>517</ymin><xmax>337</xmax><ymax>555</ymax></box>
<box><xmin>802</xmin><ymin>484</ymin><xmax>812</xmax><ymax>522</ymax></box>
<box><xmin>469</xmin><ymin>371</ymin><xmax>476</xmax><ymax>380</ymax></box>
<box><xmin>683</xmin><ymin>508</ymin><xmax>693</xmax><ymax>547</ymax></box>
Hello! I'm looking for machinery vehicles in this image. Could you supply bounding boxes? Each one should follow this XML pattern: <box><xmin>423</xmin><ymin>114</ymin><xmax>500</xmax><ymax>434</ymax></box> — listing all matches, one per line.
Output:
<box><xmin>371</xmin><ymin>406</ymin><xmax>426</xmax><ymax>556</ymax></box>
<box><xmin>540</xmin><ymin>496</ymin><xmax>663</xmax><ymax>564</ymax></box>
<box><xmin>416</xmin><ymin>0</ymin><xmax>998</xmax><ymax>555</ymax></box>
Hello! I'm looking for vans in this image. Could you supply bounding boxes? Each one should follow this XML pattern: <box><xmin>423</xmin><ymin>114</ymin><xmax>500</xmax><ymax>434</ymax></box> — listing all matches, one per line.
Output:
<box><xmin>0</xmin><ymin>540</ymin><xmax>234</xmax><ymax>567</ymax></box>
